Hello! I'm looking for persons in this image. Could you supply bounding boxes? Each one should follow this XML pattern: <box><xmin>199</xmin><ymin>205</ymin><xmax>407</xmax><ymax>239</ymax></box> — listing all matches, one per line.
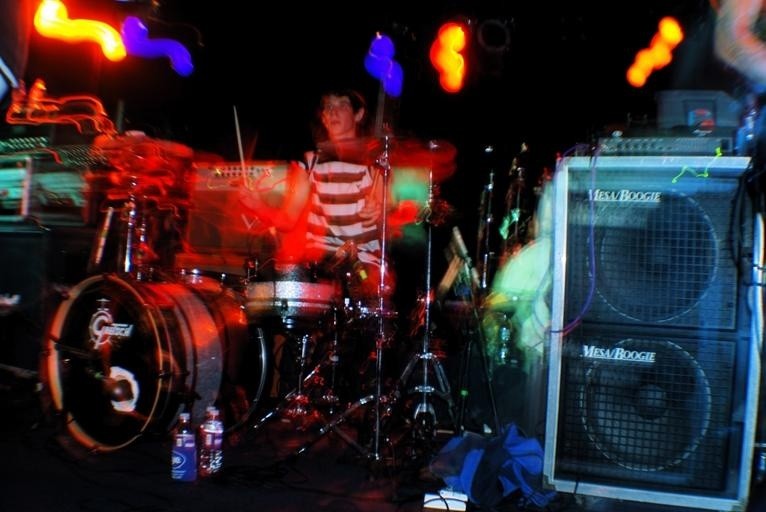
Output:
<box><xmin>713</xmin><ymin>0</ymin><xmax>766</xmax><ymax>98</ymax></box>
<box><xmin>273</xmin><ymin>83</ymin><xmax>400</xmax><ymax>423</ymax></box>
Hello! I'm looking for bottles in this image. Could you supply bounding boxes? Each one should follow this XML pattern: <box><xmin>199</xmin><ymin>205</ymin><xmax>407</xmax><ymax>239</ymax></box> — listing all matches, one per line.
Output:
<box><xmin>171</xmin><ymin>412</ymin><xmax>198</xmax><ymax>481</ymax></box>
<box><xmin>197</xmin><ymin>405</ymin><xmax>216</xmax><ymax>464</ymax></box>
<box><xmin>200</xmin><ymin>409</ymin><xmax>224</xmax><ymax>475</ymax></box>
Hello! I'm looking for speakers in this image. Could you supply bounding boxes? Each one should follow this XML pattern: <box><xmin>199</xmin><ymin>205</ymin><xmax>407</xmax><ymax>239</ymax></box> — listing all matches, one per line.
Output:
<box><xmin>547</xmin><ymin>156</ymin><xmax>762</xmax><ymax>512</ymax></box>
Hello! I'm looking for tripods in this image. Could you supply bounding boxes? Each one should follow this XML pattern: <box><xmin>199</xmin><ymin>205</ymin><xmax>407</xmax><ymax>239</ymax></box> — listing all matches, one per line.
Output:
<box><xmin>366</xmin><ymin>170</ymin><xmax>465</xmax><ymax>462</ymax></box>
<box><xmin>487</xmin><ymin>166</ymin><xmax>525</xmax><ymax>382</ymax></box>
<box><xmin>278</xmin><ymin>159</ymin><xmax>436</xmax><ymax>463</ymax></box>
<box><xmin>454</xmin><ymin>168</ymin><xmax>506</xmax><ymax>448</ymax></box>
<box><xmin>245</xmin><ymin>316</ymin><xmax>369</xmax><ymax>460</ymax></box>
<box><xmin>310</xmin><ymin>303</ymin><xmax>363</xmax><ymax>413</ymax></box>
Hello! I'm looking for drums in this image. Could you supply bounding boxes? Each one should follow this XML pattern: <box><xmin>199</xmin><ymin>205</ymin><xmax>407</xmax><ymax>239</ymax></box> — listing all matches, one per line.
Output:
<box><xmin>172</xmin><ymin>254</ymin><xmax>249</xmax><ymax>300</ymax></box>
<box><xmin>343</xmin><ymin>307</ymin><xmax>400</xmax><ymax>338</ymax></box>
<box><xmin>244</xmin><ymin>278</ymin><xmax>336</xmax><ymax>336</ymax></box>
<box><xmin>45</xmin><ymin>275</ymin><xmax>267</xmax><ymax>454</ymax></box>
